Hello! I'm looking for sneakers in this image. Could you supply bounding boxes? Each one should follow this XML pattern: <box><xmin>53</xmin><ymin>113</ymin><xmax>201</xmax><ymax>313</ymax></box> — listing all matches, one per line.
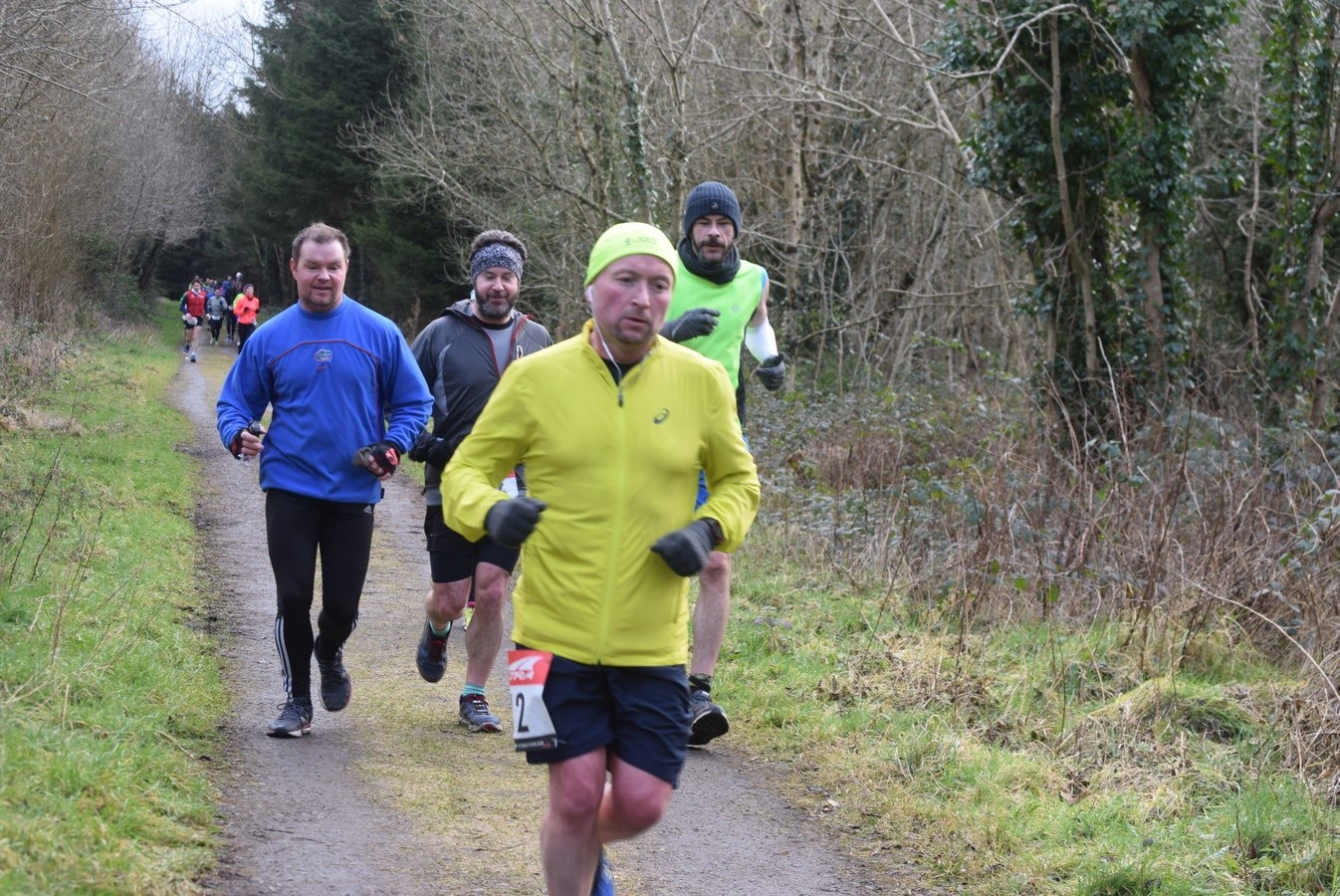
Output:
<box><xmin>458</xmin><ymin>693</ymin><xmax>501</xmax><ymax>732</ymax></box>
<box><xmin>416</xmin><ymin>613</ymin><xmax>458</xmax><ymax>682</ymax></box>
<box><xmin>686</xmin><ymin>675</ymin><xmax>731</xmax><ymax>745</ymax></box>
<box><xmin>266</xmin><ymin>697</ymin><xmax>313</xmax><ymax>738</ymax></box>
<box><xmin>315</xmin><ymin>635</ymin><xmax>351</xmax><ymax>712</ymax></box>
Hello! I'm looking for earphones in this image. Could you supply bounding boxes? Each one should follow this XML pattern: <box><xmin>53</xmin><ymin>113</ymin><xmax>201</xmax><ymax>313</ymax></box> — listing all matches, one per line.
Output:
<box><xmin>587</xmin><ymin>285</ymin><xmax>593</xmax><ymax>302</ymax></box>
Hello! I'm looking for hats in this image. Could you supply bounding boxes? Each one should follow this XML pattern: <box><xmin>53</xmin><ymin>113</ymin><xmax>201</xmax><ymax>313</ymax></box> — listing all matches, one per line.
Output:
<box><xmin>682</xmin><ymin>182</ymin><xmax>741</xmax><ymax>241</ymax></box>
<box><xmin>583</xmin><ymin>220</ymin><xmax>676</xmax><ymax>292</ymax></box>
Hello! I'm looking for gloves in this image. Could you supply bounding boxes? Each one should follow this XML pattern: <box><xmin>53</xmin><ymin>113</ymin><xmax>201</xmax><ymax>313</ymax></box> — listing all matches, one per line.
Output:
<box><xmin>672</xmin><ymin>308</ymin><xmax>720</xmax><ymax>342</ymax></box>
<box><xmin>486</xmin><ymin>499</ymin><xmax>547</xmax><ymax>551</ymax></box>
<box><xmin>244</xmin><ymin>307</ymin><xmax>248</xmax><ymax>312</ymax></box>
<box><xmin>255</xmin><ymin>309</ymin><xmax>259</xmax><ymax>314</ymax></box>
<box><xmin>652</xmin><ymin>522</ymin><xmax>714</xmax><ymax>578</ymax></box>
<box><xmin>427</xmin><ymin>438</ymin><xmax>455</xmax><ymax>468</ymax></box>
<box><xmin>754</xmin><ymin>352</ymin><xmax>785</xmax><ymax>391</ymax></box>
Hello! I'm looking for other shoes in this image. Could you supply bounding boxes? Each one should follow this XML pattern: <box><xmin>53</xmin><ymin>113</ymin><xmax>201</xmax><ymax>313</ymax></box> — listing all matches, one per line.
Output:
<box><xmin>184</xmin><ymin>342</ymin><xmax>189</xmax><ymax>353</ymax></box>
<box><xmin>227</xmin><ymin>338</ymin><xmax>230</xmax><ymax>342</ymax></box>
<box><xmin>215</xmin><ymin>341</ymin><xmax>219</xmax><ymax>346</ymax></box>
<box><xmin>190</xmin><ymin>355</ymin><xmax>195</xmax><ymax>363</ymax></box>
<box><xmin>210</xmin><ymin>336</ymin><xmax>214</xmax><ymax>344</ymax></box>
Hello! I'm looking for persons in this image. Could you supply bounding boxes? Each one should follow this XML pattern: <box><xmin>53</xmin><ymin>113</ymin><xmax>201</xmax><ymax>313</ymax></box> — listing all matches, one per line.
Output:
<box><xmin>636</xmin><ymin>181</ymin><xmax>787</xmax><ymax>745</ymax></box>
<box><xmin>398</xmin><ymin>230</ymin><xmax>558</xmax><ymax>733</ymax></box>
<box><xmin>461</xmin><ymin>588</ymin><xmax>479</xmax><ymax>632</ymax></box>
<box><xmin>218</xmin><ymin>222</ymin><xmax>435</xmax><ymax>740</ymax></box>
<box><xmin>438</xmin><ymin>217</ymin><xmax>763</xmax><ymax>896</ymax></box>
<box><xmin>179</xmin><ymin>270</ymin><xmax>259</xmax><ymax>363</ymax></box>
